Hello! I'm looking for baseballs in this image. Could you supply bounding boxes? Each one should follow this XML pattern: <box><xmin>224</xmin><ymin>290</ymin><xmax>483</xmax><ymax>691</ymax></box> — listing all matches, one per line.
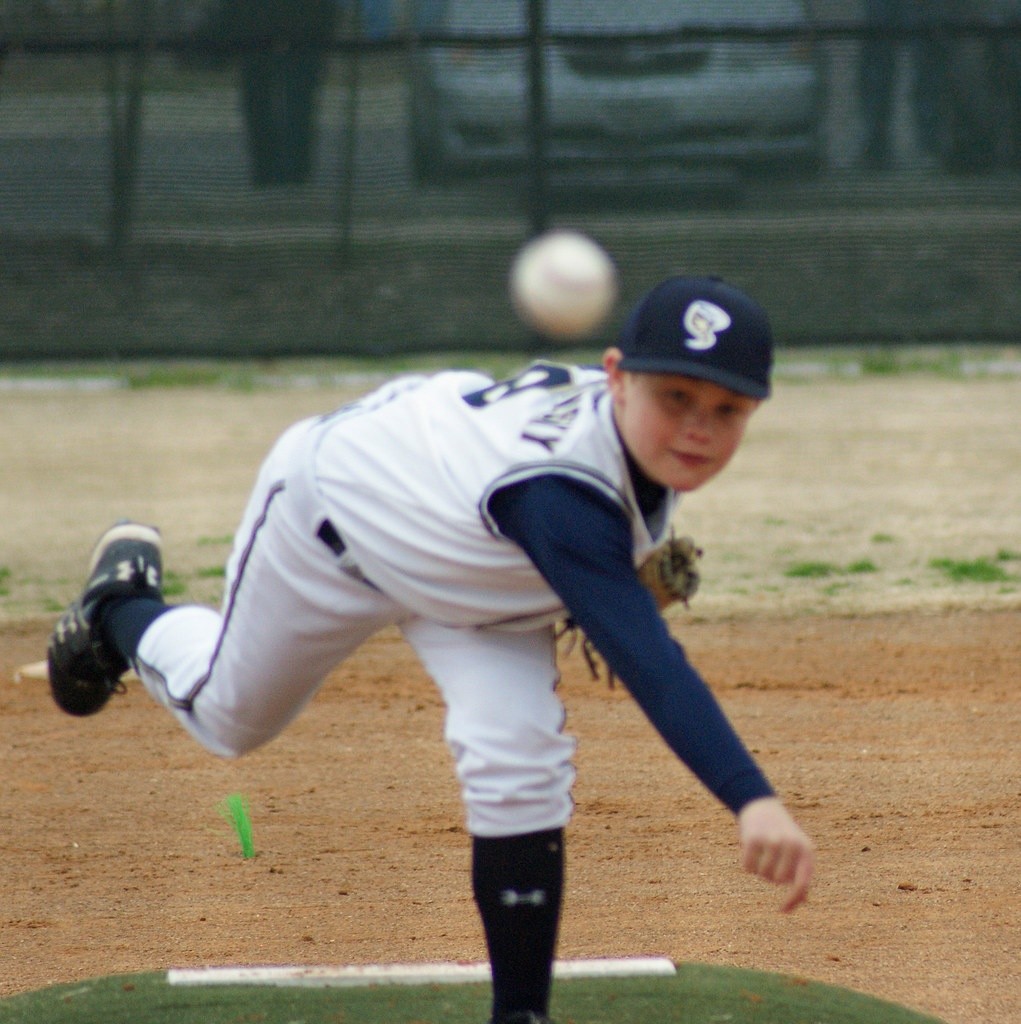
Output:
<box><xmin>511</xmin><ymin>227</ymin><xmax>623</xmax><ymax>341</ymax></box>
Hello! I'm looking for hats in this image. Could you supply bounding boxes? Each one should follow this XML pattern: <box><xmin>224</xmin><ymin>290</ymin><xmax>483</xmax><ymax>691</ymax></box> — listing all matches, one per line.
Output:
<box><xmin>616</xmin><ymin>272</ymin><xmax>773</xmax><ymax>406</ymax></box>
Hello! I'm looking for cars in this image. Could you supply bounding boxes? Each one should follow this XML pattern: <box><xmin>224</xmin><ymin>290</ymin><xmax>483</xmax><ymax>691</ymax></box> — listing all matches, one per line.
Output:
<box><xmin>382</xmin><ymin>0</ymin><xmax>838</xmax><ymax>189</ymax></box>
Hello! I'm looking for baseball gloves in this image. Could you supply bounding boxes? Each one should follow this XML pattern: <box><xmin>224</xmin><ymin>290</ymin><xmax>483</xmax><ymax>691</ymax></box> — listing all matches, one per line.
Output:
<box><xmin>634</xmin><ymin>531</ymin><xmax>702</xmax><ymax>611</ymax></box>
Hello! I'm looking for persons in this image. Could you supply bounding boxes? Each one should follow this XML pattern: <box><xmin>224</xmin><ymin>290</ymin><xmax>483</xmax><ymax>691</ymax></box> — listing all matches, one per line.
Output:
<box><xmin>216</xmin><ymin>0</ymin><xmax>340</xmax><ymax>189</ymax></box>
<box><xmin>47</xmin><ymin>275</ymin><xmax>814</xmax><ymax>1024</ymax></box>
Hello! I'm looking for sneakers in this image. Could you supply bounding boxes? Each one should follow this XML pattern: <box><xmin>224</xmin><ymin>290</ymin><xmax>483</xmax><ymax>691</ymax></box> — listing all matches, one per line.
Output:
<box><xmin>46</xmin><ymin>523</ymin><xmax>164</xmax><ymax>718</ymax></box>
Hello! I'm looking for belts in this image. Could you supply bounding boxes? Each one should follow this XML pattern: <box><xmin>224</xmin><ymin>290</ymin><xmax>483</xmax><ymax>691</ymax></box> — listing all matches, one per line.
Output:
<box><xmin>314</xmin><ymin>515</ymin><xmax>388</xmax><ymax>596</ymax></box>
